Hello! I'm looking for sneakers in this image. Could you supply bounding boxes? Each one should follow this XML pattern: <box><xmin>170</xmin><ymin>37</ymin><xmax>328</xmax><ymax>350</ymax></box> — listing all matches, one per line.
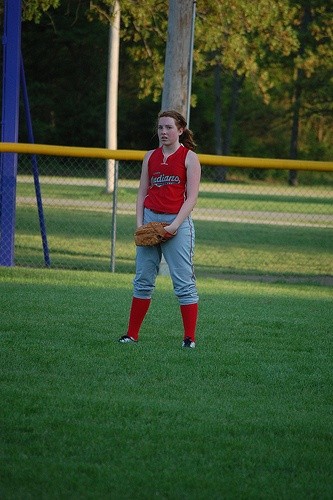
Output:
<box><xmin>181</xmin><ymin>338</ymin><xmax>195</xmax><ymax>349</ymax></box>
<box><xmin>117</xmin><ymin>336</ymin><xmax>138</xmax><ymax>344</ymax></box>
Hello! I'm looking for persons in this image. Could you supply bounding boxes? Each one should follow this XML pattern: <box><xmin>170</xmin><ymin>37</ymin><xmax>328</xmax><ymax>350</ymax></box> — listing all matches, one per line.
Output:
<box><xmin>117</xmin><ymin>111</ymin><xmax>200</xmax><ymax>349</ymax></box>
